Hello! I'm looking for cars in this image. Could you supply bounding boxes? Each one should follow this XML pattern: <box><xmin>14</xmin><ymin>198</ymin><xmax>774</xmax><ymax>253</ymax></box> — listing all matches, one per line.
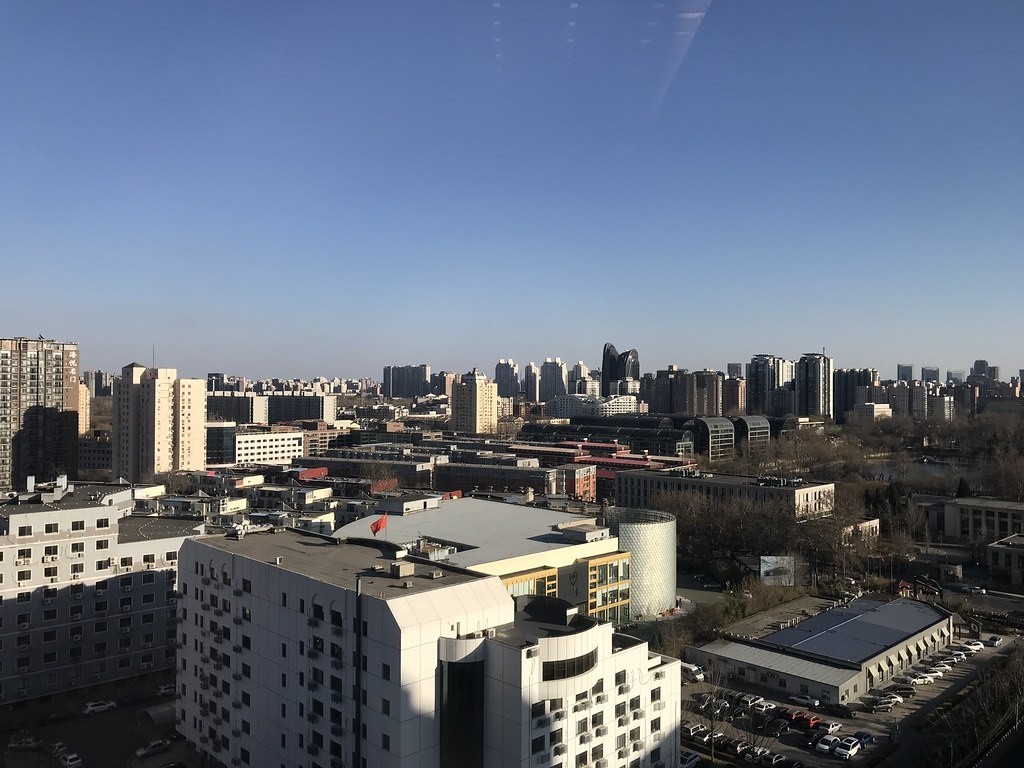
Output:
<box><xmin>955</xmin><ymin>645</ymin><xmax>977</xmax><ymax>657</ymax></box>
<box><xmin>882</xmin><ymin>694</ymin><xmax>903</xmax><ymax>705</ymax></box>
<box><xmin>929</xmin><ymin>663</ymin><xmax>952</xmax><ymax>673</ymax></box>
<box><xmin>6</xmin><ymin>727</ymin><xmax>82</xmax><ymax>768</ymax></box>
<box><xmin>939</xmin><ymin>657</ymin><xmax>957</xmax><ymax>667</ymax></box>
<box><xmin>82</xmin><ymin>700</ymin><xmax>117</xmax><ymax>715</ymax></box>
<box><xmin>155</xmin><ymin>683</ymin><xmax>176</xmax><ymax>696</ymax></box>
<box><xmin>889</xmin><ymin>686</ymin><xmax>916</xmax><ymax>698</ymax></box>
<box><xmin>799</xmin><ymin>726</ymin><xmax>828</xmax><ymax>751</ymax></box>
<box><xmin>911</xmin><ymin>675</ymin><xmax>934</xmax><ymax>686</ymax></box>
<box><xmin>822</xmin><ymin>704</ymin><xmax>856</xmax><ymax>719</ymax></box>
<box><xmin>136</xmin><ymin>740</ymin><xmax>172</xmax><ymax>759</ymax></box>
<box><xmin>964</xmin><ymin>640</ymin><xmax>984</xmax><ymax>652</ymax></box>
<box><xmin>921</xmin><ymin>669</ymin><xmax>943</xmax><ymax>679</ymax></box>
<box><xmin>853</xmin><ymin>731</ymin><xmax>876</xmax><ymax>749</ymax></box>
<box><xmin>816</xmin><ymin>735</ymin><xmax>840</xmax><ymax>754</ymax></box>
<box><xmin>870</xmin><ymin>700</ymin><xmax>894</xmax><ymax>713</ymax></box>
<box><xmin>799</xmin><ymin>713</ymin><xmax>822</xmax><ymax>731</ymax></box>
<box><xmin>790</xmin><ymin>695</ymin><xmax>819</xmax><ymax>707</ymax></box>
<box><xmin>818</xmin><ymin>720</ymin><xmax>843</xmax><ymax>735</ymax></box>
<box><xmin>161</xmin><ymin>761</ymin><xmax>187</xmax><ymax>768</ymax></box>
<box><xmin>680</xmin><ymin>661</ymin><xmax>804</xmax><ymax>768</ymax></box>
<box><xmin>950</xmin><ymin>652</ymin><xmax>966</xmax><ymax>662</ymax></box>
<box><xmin>765</xmin><ymin>719</ymin><xmax>790</xmax><ymax>738</ymax></box>
<box><xmin>987</xmin><ymin>635</ymin><xmax>1002</xmax><ymax>647</ymax></box>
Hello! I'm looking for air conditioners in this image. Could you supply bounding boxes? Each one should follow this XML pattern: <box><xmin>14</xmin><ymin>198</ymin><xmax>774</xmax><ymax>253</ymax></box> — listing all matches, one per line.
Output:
<box><xmin>123</xmin><ymin>627</ymin><xmax>131</xmax><ymax>633</ymax></box>
<box><xmin>19</xmin><ymin>689</ymin><xmax>27</xmax><ymax>696</ymax></box>
<box><xmin>473</xmin><ymin>632</ymin><xmax>483</xmax><ymax>639</ymax></box>
<box><xmin>110</xmin><ymin>557</ymin><xmax>117</xmax><ymax>565</ymax></box>
<box><xmin>123</xmin><ymin>605</ymin><xmax>130</xmax><ymax>611</ymax></box>
<box><xmin>527</xmin><ymin>649</ymin><xmax>538</xmax><ymax>658</ymax></box>
<box><xmin>44</xmin><ymin>598</ymin><xmax>51</xmax><ymax>604</ymax></box>
<box><xmin>307</xmin><ymin>618</ymin><xmax>342</xmax><ymax>768</ymax></box>
<box><xmin>20</xmin><ymin>645</ymin><xmax>28</xmax><ymax>651</ymax></box>
<box><xmin>275</xmin><ymin>556</ymin><xmax>284</xmax><ymax>564</ymax></box>
<box><xmin>539</xmin><ymin>671</ymin><xmax>666</xmax><ymax>768</ymax></box>
<box><xmin>149</xmin><ymin>563</ymin><xmax>155</xmax><ymax>569</ymax></box>
<box><xmin>53</xmin><ymin>578</ymin><xmax>57</xmax><ymax>583</ymax></box>
<box><xmin>76</xmin><ymin>593</ymin><xmax>82</xmax><ymax>599</ymax></box>
<box><xmin>169</xmin><ymin>561</ymin><xmax>242</xmax><ymax>767</ymax></box>
<box><xmin>97</xmin><ymin>590</ymin><xmax>102</xmax><ymax>596</ymax></box>
<box><xmin>20</xmin><ymin>582</ymin><xmax>27</xmax><ymax>586</ymax></box>
<box><xmin>73</xmin><ymin>635</ymin><xmax>80</xmax><ymax>641</ymax></box>
<box><xmin>21</xmin><ymin>623</ymin><xmax>28</xmax><ymax>629</ymax></box>
<box><xmin>168</xmin><ymin>656</ymin><xmax>175</xmax><ymax>663</ymax></box>
<box><xmin>16</xmin><ymin>558</ymin><xmax>32</xmax><ymax>565</ymax></box>
<box><xmin>79</xmin><ymin>553</ymin><xmax>84</xmax><ymax>557</ymax></box>
<box><xmin>123</xmin><ymin>648</ymin><xmax>129</xmax><ymax>652</ymax></box>
<box><xmin>44</xmin><ymin>555</ymin><xmax>58</xmax><ymax>563</ymax></box>
<box><xmin>145</xmin><ymin>643</ymin><xmax>152</xmax><ymax>648</ymax></box>
<box><xmin>74</xmin><ymin>614</ymin><xmax>81</xmax><ymax>620</ymax></box>
<box><xmin>128</xmin><ymin>567</ymin><xmax>133</xmax><ymax>572</ymax></box>
<box><xmin>125</xmin><ymin>586</ymin><xmax>131</xmax><ymax>591</ymax></box>
<box><xmin>486</xmin><ymin>629</ymin><xmax>495</xmax><ymax>638</ymax></box>
<box><xmin>20</xmin><ymin>666</ymin><xmax>28</xmax><ymax>673</ymax></box>
<box><xmin>75</xmin><ymin>574</ymin><xmax>80</xmax><ymax>579</ymax></box>
<box><xmin>168</xmin><ymin>639</ymin><xmax>176</xmax><ymax>645</ymax></box>
<box><xmin>140</xmin><ymin>663</ymin><xmax>154</xmax><ymax>668</ymax></box>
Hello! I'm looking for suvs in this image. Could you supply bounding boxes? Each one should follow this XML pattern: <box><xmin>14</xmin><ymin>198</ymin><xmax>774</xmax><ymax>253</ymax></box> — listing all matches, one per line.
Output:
<box><xmin>834</xmin><ymin>737</ymin><xmax>861</xmax><ymax>760</ymax></box>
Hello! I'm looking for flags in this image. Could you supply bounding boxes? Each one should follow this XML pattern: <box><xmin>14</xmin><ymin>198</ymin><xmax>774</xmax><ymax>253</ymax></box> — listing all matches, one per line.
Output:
<box><xmin>369</xmin><ymin>513</ymin><xmax>385</xmax><ymax>538</ymax></box>
